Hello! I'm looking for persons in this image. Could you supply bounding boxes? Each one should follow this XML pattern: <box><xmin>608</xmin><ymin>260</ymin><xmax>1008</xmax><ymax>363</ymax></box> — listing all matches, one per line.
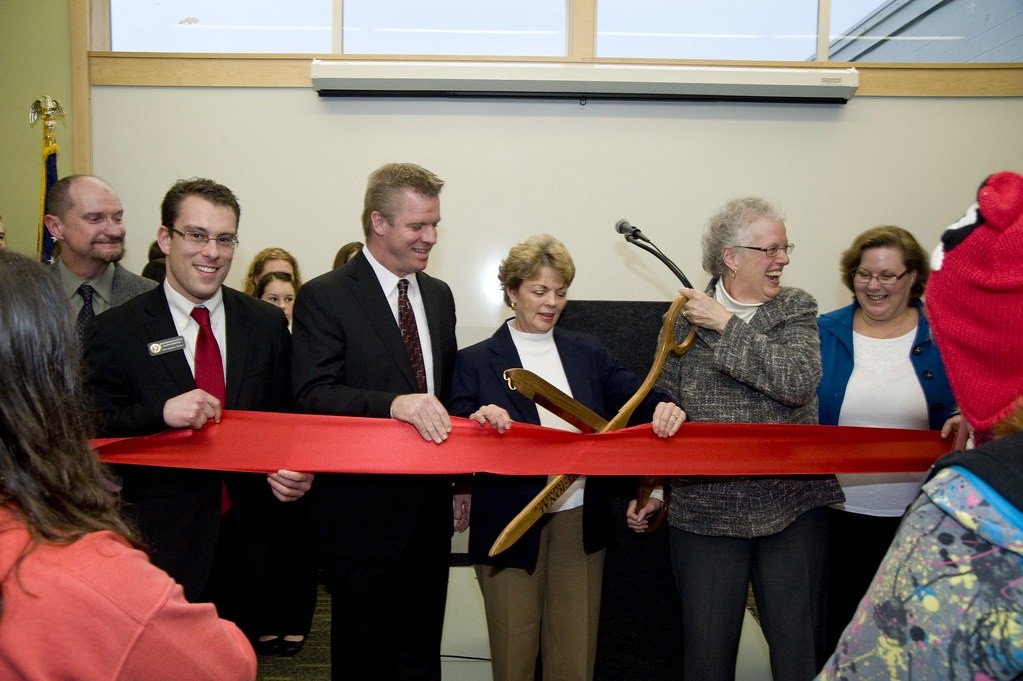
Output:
<box><xmin>245</xmin><ymin>247</ymin><xmax>320</xmax><ymax>658</ymax></box>
<box><xmin>141</xmin><ymin>239</ymin><xmax>168</xmax><ymax>283</ymax></box>
<box><xmin>818</xmin><ymin>223</ymin><xmax>975</xmax><ymax>654</ymax></box>
<box><xmin>450</xmin><ymin>238</ymin><xmax>689</xmax><ymax>681</ymax></box>
<box><xmin>626</xmin><ymin>196</ymin><xmax>848</xmax><ymax>681</ymax></box>
<box><xmin>84</xmin><ymin>176</ymin><xmax>316</xmax><ymax>654</ymax></box>
<box><xmin>0</xmin><ymin>249</ymin><xmax>257</xmax><ymax>681</ymax></box>
<box><xmin>35</xmin><ymin>174</ymin><xmax>160</xmax><ymax>432</ymax></box>
<box><xmin>809</xmin><ymin>172</ymin><xmax>1023</xmax><ymax>681</ymax></box>
<box><xmin>334</xmin><ymin>242</ymin><xmax>365</xmax><ymax>271</ymax></box>
<box><xmin>288</xmin><ymin>163</ymin><xmax>476</xmax><ymax>681</ymax></box>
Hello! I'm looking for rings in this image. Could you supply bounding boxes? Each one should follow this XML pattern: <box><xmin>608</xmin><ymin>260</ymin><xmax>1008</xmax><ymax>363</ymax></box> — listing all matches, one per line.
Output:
<box><xmin>671</xmin><ymin>412</ymin><xmax>678</xmax><ymax>419</ymax></box>
<box><xmin>682</xmin><ymin>311</ymin><xmax>686</xmax><ymax>317</ymax></box>
<box><xmin>969</xmin><ymin>432</ymin><xmax>974</xmax><ymax>434</ymax></box>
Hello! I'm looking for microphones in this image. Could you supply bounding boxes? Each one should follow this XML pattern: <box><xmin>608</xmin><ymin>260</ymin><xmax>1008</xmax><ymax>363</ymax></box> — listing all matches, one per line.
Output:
<box><xmin>615</xmin><ymin>219</ymin><xmax>650</xmax><ymax>242</ymax></box>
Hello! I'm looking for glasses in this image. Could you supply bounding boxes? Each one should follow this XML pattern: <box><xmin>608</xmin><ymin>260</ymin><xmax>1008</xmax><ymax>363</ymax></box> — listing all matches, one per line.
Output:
<box><xmin>165</xmin><ymin>227</ymin><xmax>239</xmax><ymax>247</ymax></box>
<box><xmin>850</xmin><ymin>266</ymin><xmax>910</xmax><ymax>285</ymax></box>
<box><xmin>730</xmin><ymin>243</ymin><xmax>795</xmax><ymax>257</ymax></box>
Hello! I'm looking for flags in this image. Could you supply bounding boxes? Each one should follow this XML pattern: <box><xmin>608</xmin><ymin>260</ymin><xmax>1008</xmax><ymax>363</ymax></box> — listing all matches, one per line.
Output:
<box><xmin>36</xmin><ymin>132</ymin><xmax>59</xmax><ymax>265</ymax></box>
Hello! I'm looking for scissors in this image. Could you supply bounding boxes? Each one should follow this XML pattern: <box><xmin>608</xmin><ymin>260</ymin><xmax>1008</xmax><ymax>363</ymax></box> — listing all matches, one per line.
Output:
<box><xmin>489</xmin><ymin>295</ymin><xmax>701</xmax><ymax>560</ymax></box>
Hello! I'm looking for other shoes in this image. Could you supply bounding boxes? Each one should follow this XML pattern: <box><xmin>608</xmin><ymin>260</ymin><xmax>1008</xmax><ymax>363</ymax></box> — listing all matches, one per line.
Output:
<box><xmin>255</xmin><ymin>634</ymin><xmax>281</xmax><ymax>656</ymax></box>
<box><xmin>279</xmin><ymin>633</ymin><xmax>308</xmax><ymax>656</ymax></box>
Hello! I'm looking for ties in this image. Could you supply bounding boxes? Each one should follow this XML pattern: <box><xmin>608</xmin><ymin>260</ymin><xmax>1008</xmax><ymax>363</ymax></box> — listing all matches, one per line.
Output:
<box><xmin>397</xmin><ymin>279</ymin><xmax>429</xmax><ymax>394</ymax></box>
<box><xmin>76</xmin><ymin>283</ymin><xmax>98</xmax><ymax>370</ymax></box>
<box><xmin>190</xmin><ymin>306</ymin><xmax>227</xmax><ymax>410</ymax></box>
